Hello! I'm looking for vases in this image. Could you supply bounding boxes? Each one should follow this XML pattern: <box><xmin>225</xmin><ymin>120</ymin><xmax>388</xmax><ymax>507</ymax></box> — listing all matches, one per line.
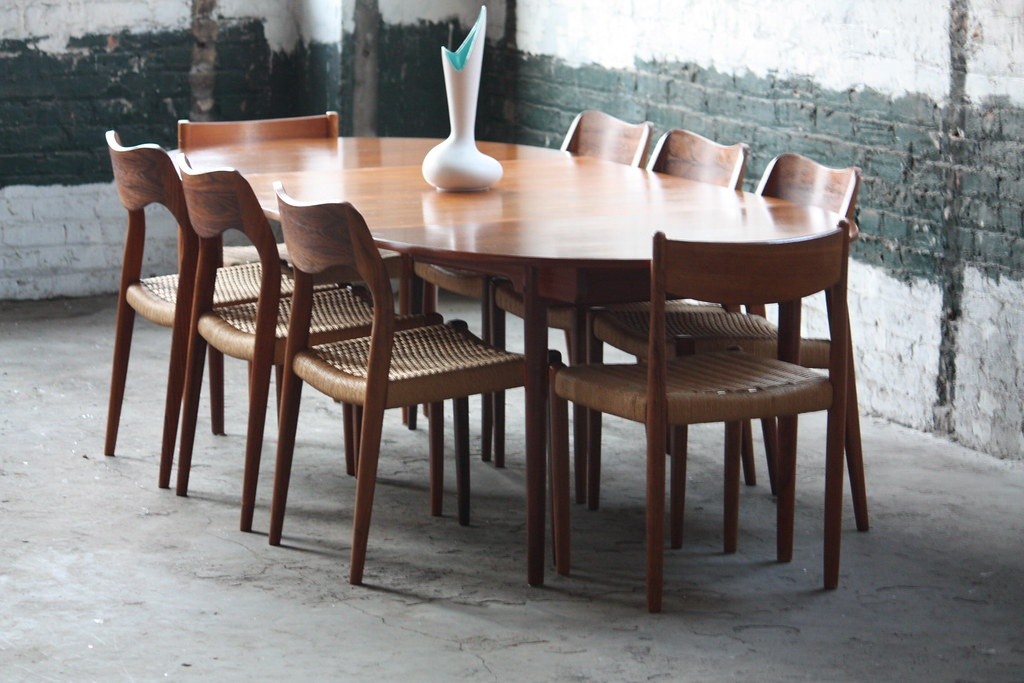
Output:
<box><xmin>421</xmin><ymin>6</ymin><xmax>504</xmax><ymax>192</ymax></box>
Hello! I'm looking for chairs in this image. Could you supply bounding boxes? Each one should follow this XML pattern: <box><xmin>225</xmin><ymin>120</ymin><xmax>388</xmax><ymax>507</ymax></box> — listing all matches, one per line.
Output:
<box><xmin>105</xmin><ymin>110</ymin><xmax>871</xmax><ymax>616</ymax></box>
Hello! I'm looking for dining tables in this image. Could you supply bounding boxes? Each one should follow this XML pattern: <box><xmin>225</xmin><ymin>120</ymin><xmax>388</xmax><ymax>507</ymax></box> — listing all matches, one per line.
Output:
<box><xmin>165</xmin><ymin>135</ymin><xmax>850</xmax><ymax>589</ymax></box>
